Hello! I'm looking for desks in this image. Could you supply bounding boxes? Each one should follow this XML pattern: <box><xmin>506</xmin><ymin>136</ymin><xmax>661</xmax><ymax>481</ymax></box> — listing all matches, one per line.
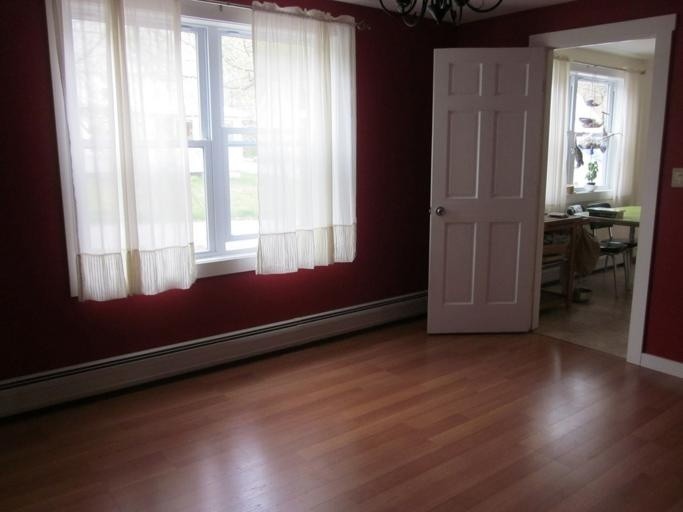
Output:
<box><xmin>541</xmin><ymin>214</ymin><xmax>591</xmax><ymax>309</ymax></box>
<box><xmin>574</xmin><ymin>205</ymin><xmax>641</xmax><ymax>267</ymax></box>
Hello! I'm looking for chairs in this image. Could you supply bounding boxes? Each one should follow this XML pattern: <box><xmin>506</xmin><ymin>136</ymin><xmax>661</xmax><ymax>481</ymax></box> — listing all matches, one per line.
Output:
<box><xmin>566</xmin><ymin>202</ymin><xmax>637</xmax><ymax>300</ymax></box>
